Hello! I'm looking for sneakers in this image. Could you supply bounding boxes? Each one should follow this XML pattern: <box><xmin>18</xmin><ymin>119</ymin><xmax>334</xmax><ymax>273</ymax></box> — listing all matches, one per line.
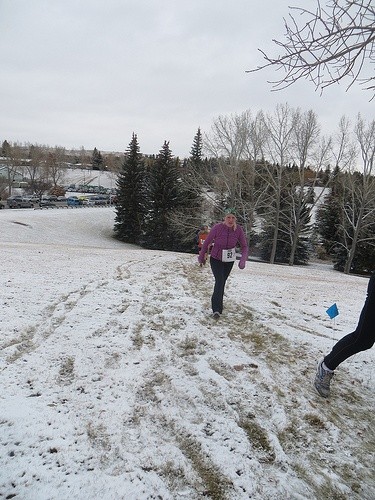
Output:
<box><xmin>213</xmin><ymin>312</ymin><xmax>220</xmax><ymax>318</ymax></box>
<box><xmin>313</xmin><ymin>359</ymin><xmax>334</xmax><ymax>398</ymax></box>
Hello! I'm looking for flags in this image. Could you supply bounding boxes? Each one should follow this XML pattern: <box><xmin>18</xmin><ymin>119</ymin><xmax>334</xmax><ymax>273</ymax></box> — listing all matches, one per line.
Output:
<box><xmin>326</xmin><ymin>303</ymin><xmax>339</xmax><ymax>319</ymax></box>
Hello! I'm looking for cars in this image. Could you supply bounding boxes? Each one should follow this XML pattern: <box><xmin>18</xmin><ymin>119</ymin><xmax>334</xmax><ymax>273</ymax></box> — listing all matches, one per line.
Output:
<box><xmin>6</xmin><ymin>184</ymin><xmax>118</xmax><ymax>209</ymax></box>
<box><xmin>0</xmin><ymin>200</ymin><xmax>6</xmax><ymax>209</ymax></box>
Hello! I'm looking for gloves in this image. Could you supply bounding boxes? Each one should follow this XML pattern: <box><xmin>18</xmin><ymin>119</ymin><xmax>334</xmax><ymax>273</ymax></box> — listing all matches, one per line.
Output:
<box><xmin>238</xmin><ymin>257</ymin><xmax>246</xmax><ymax>269</ymax></box>
<box><xmin>198</xmin><ymin>251</ymin><xmax>204</xmax><ymax>262</ymax></box>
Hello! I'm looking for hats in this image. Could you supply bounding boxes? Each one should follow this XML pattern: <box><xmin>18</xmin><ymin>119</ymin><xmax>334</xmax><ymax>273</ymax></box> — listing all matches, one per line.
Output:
<box><xmin>225</xmin><ymin>208</ymin><xmax>237</xmax><ymax>216</ymax></box>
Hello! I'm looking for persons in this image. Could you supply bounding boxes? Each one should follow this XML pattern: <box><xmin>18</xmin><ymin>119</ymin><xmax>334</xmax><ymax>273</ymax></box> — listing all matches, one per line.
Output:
<box><xmin>316</xmin><ymin>271</ymin><xmax>375</xmax><ymax>395</ymax></box>
<box><xmin>198</xmin><ymin>209</ymin><xmax>248</xmax><ymax>316</ymax></box>
